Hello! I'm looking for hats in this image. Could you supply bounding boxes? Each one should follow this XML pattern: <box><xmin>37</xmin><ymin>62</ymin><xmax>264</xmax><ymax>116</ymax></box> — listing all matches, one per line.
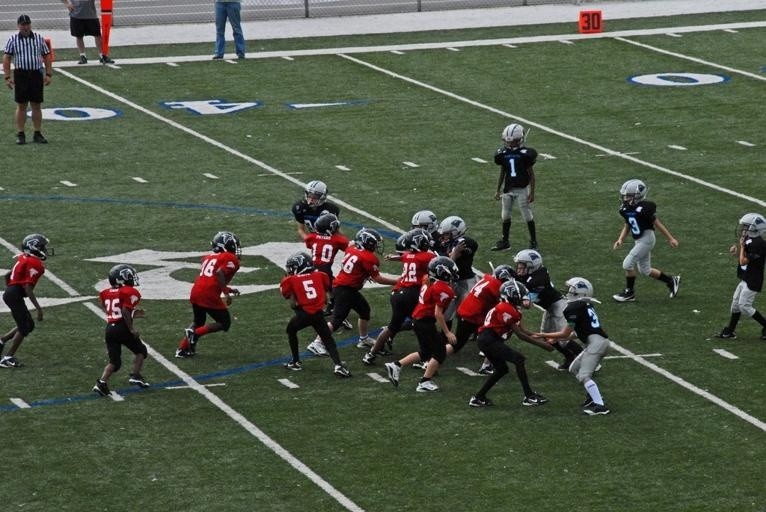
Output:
<box><xmin>17</xmin><ymin>15</ymin><xmax>31</xmax><ymax>25</ymax></box>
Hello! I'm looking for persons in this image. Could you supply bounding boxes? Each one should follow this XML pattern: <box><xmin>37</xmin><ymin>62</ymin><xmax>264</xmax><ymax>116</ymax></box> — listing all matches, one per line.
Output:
<box><xmin>492</xmin><ymin>122</ymin><xmax>540</xmax><ymax>251</ymax></box>
<box><xmin>211</xmin><ymin>1</ymin><xmax>246</xmax><ymax>60</ymax></box>
<box><xmin>61</xmin><ymin>0</ymin><xmax>115</xmax><ymax>64</ymax></box>
<box><xmin>279</xmin><ymin>177</ymin><xmax>611</xmax><ymax>415</ymax></box>
<box><xmin>171</xmin><ymin>230</ymin><xmax>242</xmax><ymax>359</ymax></box>
<box><xmin>89</xmin><ymin>262</ymin><xmax>150</xmax><ymax>396</ymax></box>
<box><xmin>613</xmin><ymin>176</ymin><xmax>684</xmax><ymax>302</ymax></box>
<box><xmin>714</xmin><ymin>211</ymin><xmax>766</xmax><ymax>344</ymax></box>
<box><xmin>0</xmin><ymin>231</ymin><xmax>51</xmax><ymax>369</ymax></box>
<box><xmin>2</xmin><ymin>14</ymin><xmax>54</xmax><ymax>146</ymax></box>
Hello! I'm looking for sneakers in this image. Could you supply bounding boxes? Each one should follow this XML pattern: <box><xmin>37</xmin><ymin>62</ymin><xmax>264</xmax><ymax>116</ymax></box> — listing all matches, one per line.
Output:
<box><xmin>78</xmin><ymin>55</ymin><xmax>87</xmax><ymax>64</ymax></box>
<box><xmin>0</xmin><ymin>358</ymin><xmax>25</xmax><ymax>368</ymax></box>
<box><xmin>92</xmin><ymin>380</ymin><xmax>112</xmax><ymax>397</ymax></box>
<box><xmin>667</xmin><ymin>276</ymin><xmax>681</xmax><ymax>299</ymax></box>
<box><xmin>129</xmin><ymin>373</ymin><xmax>151</xmax><ymax>388</ymax></box>
<box><xmin>522</xmin><ymin>392</ymin><xmax>548</xmax><ymax>406</ymax></box>
<box><xmin>100</xmin><ymin>55</ymin><xmax>114</xmax><ymax>63</ymax></box>
<box><xmin>529</xmin><ymin>240</ymin><xmax>537</xmax><ymax>249</ymax></box>
<box><xmin>175</xmin><ymin>328</ymin><xmax>197</xmax><ymax>358</ymax></box>
<box><xmin>582</xmin><ymin>393</ymin><xmax>610</xmax><ymax>416</ymax></box>
<box><xmin>490</xmin><ymin>240</ymin><xmax>511</xmax><ymax>252</ymax></box>
<box><xmin>16</xmin><ymin>134</ymin><xmax>25</xmax><ymax>144</ymax></box>
<box><xmin>479</xmin><ymin>363</ymin><xmax>494</xmax><ymax>374</ymax></box>
<box><xmin>34</xmin><ymin>134</ymin><xmax>47</xmax><ymax>144</ymax></box>
<box><xmin>613</xmin><ymin>288</ymin><xmax>636</xmax><ymax>302</ymax></box>
<box><xmin>469</xmin><ymin>393</ymin><xmax>493</xmax><ymax>407</ymax></box>
<box><xmin>283</xmin><ymin>318</ymin><xmax>440</xmax><ymax>394</ymax></box>
<box><xmin>711</xmin><ymin>327</ymin><xmax>737</xmax><ymax>340</ymax></box>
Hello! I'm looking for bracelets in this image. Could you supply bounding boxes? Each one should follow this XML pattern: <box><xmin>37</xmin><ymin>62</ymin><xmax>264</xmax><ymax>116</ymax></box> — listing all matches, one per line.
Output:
<box><xmin>45</xmin><ymin>73</ymin><xmax>52</xmax><ymax>79</ymax></box>
<box><xmin>4</xmin><ymin>75</ymin><xmax>10</xmax><ymax>80</ymax></box>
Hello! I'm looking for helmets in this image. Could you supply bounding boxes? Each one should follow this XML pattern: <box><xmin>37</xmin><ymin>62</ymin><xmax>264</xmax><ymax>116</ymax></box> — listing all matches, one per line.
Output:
<box><xmin>560</xmin><ymin>276</ymin><xmax>594</xmax><ymax>300</ymax></box>
<box><xmin>395</xmin><ymin>210</ymin><xmax>467</xmax><ymax>284</ymax></box>
<box><xmin>501</xmin><ymin>124</ymin><xmax>526</xmax><ymax>151</ymax></box>
<box><xmin>212</xmin><ymin>231</ymin><xmax>242</xmax><ymax>261</ymax></box>
<box><xmin>21</xmin><ymin>234</ymin><xmax>50</xmax><ymax>261</ymax></box>
<box><xmin>304</xmin><ymin>180</ymin><xmax>384</xmax><ymax>256</ymax></box>
<box><xmin>494</xmin><ymin>249</ymin><xmax>543</xmax><ymax>309</ymax></box>
<box><xmin>286</xmin><ymin>253</ymin><xmax>314</xmax><ymax>275</ymax></box>
<box><xmin>109</xmin><ymin>264</ymin><xmax>140</xmax><ymax>289</ymax></box>
<box><xmin>619</xmin><ymin>179</ymin><xmax>648</xmax><ymax>205</ymax></box>
<box><xmin>735</xmin><ymin>213</ymin><xmax>766</xmax><ymax>238</ymax></box>
